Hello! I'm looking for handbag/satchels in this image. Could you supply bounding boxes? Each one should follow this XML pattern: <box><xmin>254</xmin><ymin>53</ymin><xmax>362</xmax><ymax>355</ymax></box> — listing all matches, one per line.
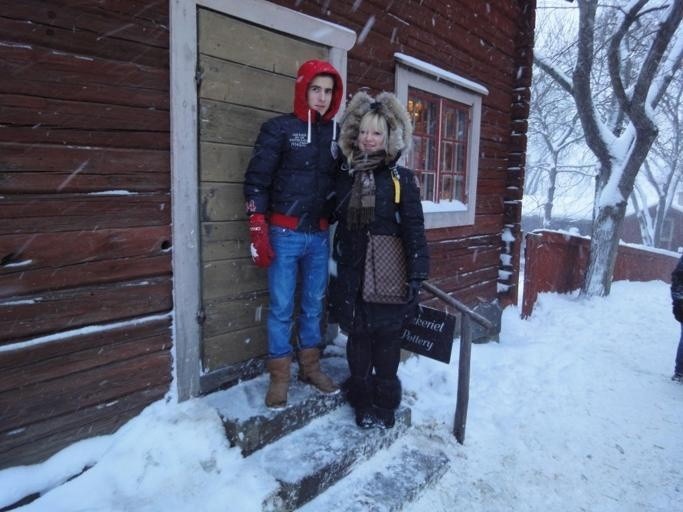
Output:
<box><xmin>361</xmin><ymin>234</ymin><xmax>409</xmax><ymax>305</ymax></box>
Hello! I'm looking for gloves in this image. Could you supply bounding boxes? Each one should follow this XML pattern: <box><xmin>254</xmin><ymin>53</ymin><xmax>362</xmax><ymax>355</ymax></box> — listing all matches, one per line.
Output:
<box><xmin>249</xmin><ymin>222</ymin><xmax>275</xmax><ymax>268</ymax></box>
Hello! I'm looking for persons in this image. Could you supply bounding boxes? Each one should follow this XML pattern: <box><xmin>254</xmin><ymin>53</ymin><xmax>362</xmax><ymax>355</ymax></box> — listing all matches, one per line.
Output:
<box><xmin>329</xmin><ymin>89</ymin><xmax>429</xmax><ymax>429</ymax></box>
<box><xmin>670</xmin><ymin>254</ymin><xmax>683</xmax><ymax>382</ymax></box>
<box><xmin>241</xmin><ymin>60</ymin><xmax>345</xmax><ymax>410</ymax></box>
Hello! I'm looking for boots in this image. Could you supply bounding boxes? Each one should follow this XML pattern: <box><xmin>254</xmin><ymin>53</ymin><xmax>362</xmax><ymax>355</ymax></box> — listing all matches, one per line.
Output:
<box><xmin>352</xmin><ymin>374</ymin><xmax>402</xmax><ymax>428</ymax></box>
<box><xmin>297</xmin><ymin>347</ymin><xmax>337</xmax><ymax>393</ymax></box>
<box><xmin>265</xmin><ymin>356</ymin><xmax>291</xmax><ymax>408</ymax></box>
